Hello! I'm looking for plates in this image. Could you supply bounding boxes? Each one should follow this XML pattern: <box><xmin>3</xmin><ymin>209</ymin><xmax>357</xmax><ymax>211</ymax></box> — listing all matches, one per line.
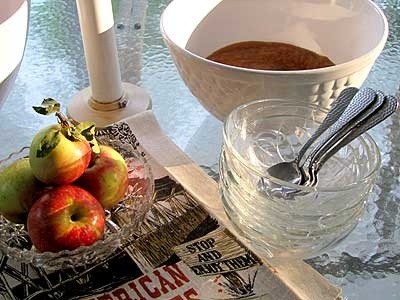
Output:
<box><xmin>218</xmin><ymin>99</ymin><xmax>381</xmax><ymax>259</ymax></box>
<box><xmin>0</xmin><ymin>122</ymin><xmax>154</xmax><ymax>270</ymax></box>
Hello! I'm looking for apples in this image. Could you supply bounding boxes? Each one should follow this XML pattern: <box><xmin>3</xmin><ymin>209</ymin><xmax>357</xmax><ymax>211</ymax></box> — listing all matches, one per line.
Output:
<box><xmin>0</xmin><ymin>158</ymin><xmax>34</xmax><ymax>224</ymax></box>
<box><xmin>26</xmin><ymin>185</ymin><xmax>105</xmax><ymax>251</ymax></box>
<box><xmin>29</xmin><ymin>123</ymin><xmax>91</xmax><ymax>186</ymax></box>
<box><xmin>75</xmin><ymin>145</ymin><xmax>129</xmax><ymax>209</ymax></box>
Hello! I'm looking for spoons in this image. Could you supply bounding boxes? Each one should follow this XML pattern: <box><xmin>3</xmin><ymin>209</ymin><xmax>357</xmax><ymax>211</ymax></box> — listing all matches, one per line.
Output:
<box><xmin>256</xmin><ymin>87</ymin><xmax>397</xmax><ymax>199</ymax></box>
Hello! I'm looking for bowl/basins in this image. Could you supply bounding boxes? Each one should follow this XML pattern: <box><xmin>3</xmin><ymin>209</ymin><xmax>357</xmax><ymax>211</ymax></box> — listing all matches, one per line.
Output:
<box><xmin>158</xmin><ymin>0</ymin><xmax>391</xmax><ymax>124</ymax></box>
